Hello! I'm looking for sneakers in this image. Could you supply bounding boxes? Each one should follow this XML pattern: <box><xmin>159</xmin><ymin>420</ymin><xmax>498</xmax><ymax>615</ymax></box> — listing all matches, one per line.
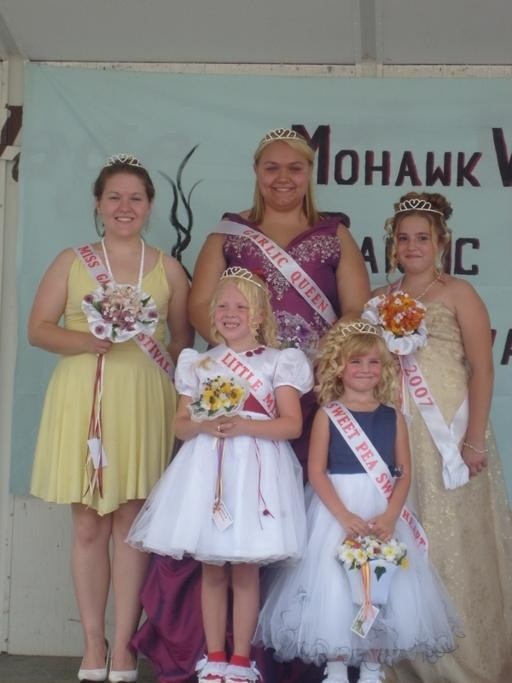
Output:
<box><xmin>356</xmin><ymin>661</ymin><xmax>386</xmax><ymax>683</ymax></box>
<box><xmin>321</xmin><ymin>660</ymin><xmax>350</xmax><ymax>683</ymax></box>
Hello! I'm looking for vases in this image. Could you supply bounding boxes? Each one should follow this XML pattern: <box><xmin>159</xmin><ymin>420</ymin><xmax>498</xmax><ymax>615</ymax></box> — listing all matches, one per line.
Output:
<box><xmin>342</xmin><ymin>559</ymin><xmax>397</xmax><ymax>607</ymax></box>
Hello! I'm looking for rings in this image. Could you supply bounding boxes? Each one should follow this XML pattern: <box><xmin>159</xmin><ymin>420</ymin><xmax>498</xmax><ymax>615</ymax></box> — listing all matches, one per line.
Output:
<box><xmin>217</xmin><ymin>426</ymin><xmax>220</xmax><ymax>432</ymax></box>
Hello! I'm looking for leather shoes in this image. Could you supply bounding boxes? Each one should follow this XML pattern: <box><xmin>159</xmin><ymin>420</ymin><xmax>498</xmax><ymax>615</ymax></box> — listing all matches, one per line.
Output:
<box><xmin>108</xmin><ymin>646</ymin><xmax>140</xmax><ymax>683</ymax></box>
<box><xmin>195</xmin><ymin>656</ymin><xmax>263</xmax><ymax>683</ymax></box>
<box><xmin>77</xmin><ymin>635</ymin><xmax>110</xmax><ymax>682</ymax></box>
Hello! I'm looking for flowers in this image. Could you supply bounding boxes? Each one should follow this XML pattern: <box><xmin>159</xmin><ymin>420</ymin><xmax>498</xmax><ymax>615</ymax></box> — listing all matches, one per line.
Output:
<box><xmin>198</xmin><ymin>371</ymin><xmax>245</xmax><ymax>416</ymax></box>
<box><xmin>336</xmin><ymin>530</ymin><xmax>409</xmax><ymax>572</ymax></box>
<box><xmin>272</xmin><ymin>309</ymin><xmax>311</xmax><ymax>351</ymax></box>
<box><xmin>91</xmin><ymin>282</ymin><xmax>146</xmax><ymax>333</ymax></box>
<box><xmin>375</xmin><ymin>291</ymin><xmax>428</xmax><ymax>339</ymax></box>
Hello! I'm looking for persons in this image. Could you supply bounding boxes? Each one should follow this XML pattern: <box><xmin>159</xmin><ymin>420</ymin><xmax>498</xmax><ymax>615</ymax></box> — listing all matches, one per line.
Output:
<box><xmin>372</xmin><ymin>194</ymin><xmax>511</xmax><ymax>683</ymax></box>
<box><xmin>251</xmin><ymin>318</ymin><xmax>466</xmax><ymax>683</ymax></box>
<box><xmin>128</xmin><ymin>127</ymin><xmax>371</xmax><ymax>683</ymax></box>
<box><xmin>126</xmin><ymin>264</ymin><xmax>321</xmax><ymax>683</ymax></box>
<box><xmin>28</xmin><ymin>155</ymin><xmax>194</xmax><ymax>683</ymax></box>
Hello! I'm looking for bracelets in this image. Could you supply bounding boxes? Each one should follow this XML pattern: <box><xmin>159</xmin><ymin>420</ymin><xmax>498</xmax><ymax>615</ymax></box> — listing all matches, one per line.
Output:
<box><xmin>462</xmin><ymin>443</ymin><xmax>489</xmax><ymax>454</ymax></box>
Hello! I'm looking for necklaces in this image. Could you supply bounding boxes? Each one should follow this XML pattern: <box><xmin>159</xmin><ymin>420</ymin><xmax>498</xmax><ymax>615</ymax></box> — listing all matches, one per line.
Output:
<box><xmin>99</xmin><ymin>237</ymin><xmax>145</xmax><ymax>293</ymax></box>
<box><xmin>399</xmin><ymin>276</ymin><xmax>440</xmax><ymax>300</ymax></box>
